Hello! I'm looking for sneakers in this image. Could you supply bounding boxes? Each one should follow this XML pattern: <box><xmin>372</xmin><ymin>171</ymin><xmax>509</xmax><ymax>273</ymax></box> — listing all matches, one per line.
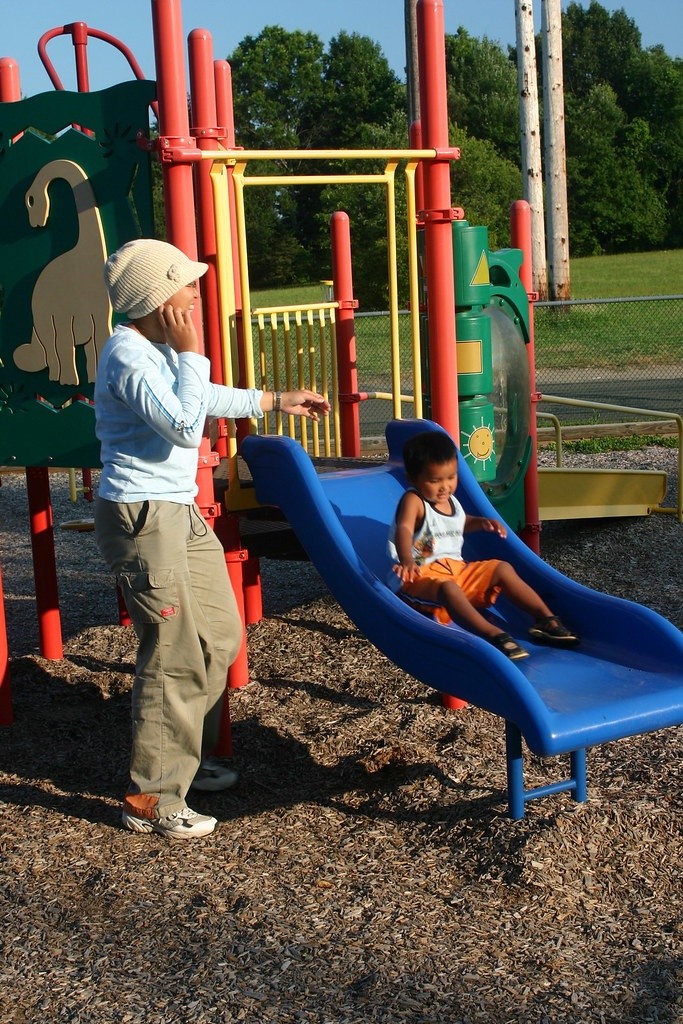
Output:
<box><xmin>191</xmin><ymin>757</ymin><xmax>237</xmax><ymax>789</ymax></box>
<box><xmin>122</xmin><ymin>803</ymin><xmax>220</xmax><ymax>839</ymax></box>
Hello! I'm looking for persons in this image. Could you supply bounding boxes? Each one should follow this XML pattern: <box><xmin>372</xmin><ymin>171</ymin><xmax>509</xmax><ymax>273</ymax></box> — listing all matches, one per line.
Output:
<box><xmin>94</xmin><ymin>239</ymin><xmax>332</xmax><ymax>840</ymax></box>
<box><xmin>386</xmin><ymin>429</ymin><xmax>578</xmax><ymax>659</ymax></box>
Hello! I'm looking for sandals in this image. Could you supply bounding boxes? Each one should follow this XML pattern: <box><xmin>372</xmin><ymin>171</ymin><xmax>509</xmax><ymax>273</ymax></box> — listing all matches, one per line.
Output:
<box><xmin>485</xmin><ymin>632</ymin><xmax>529</xmax><ymax>659</ymax></box>
<box><xmin>527</xmin><ymin>613</ymin><xmax>578</xmax><ymax>644</ymax></box>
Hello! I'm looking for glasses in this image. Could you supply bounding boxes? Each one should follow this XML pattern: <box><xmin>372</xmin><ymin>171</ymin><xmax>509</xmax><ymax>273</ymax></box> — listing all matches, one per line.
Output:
<box><xmin>185</xmin><ymin>281</ymin><xmax>198</xmax><ymax>288</ymax></box>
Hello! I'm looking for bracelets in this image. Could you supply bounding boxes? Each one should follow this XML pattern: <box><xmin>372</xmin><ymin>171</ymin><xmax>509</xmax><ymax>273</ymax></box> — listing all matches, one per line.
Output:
<box><xmin>272</xmin><ymin>390</ymin><xmax>281</xmax><ymax>412</ymax></box>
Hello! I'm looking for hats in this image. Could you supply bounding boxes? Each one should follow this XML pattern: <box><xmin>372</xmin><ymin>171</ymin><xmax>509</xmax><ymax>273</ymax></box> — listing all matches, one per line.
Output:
<box><xmin>103</xmin><ymin>239</ymin><xmax>209</xmax><ymax>318</ymax></box>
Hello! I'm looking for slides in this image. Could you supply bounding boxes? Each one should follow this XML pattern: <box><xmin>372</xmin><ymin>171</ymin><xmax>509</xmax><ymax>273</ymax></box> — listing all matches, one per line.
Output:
<box><xmin>241</xmin><ymin>417</ymin><xmax>683</xmax><ymax>758</ymax></box>
